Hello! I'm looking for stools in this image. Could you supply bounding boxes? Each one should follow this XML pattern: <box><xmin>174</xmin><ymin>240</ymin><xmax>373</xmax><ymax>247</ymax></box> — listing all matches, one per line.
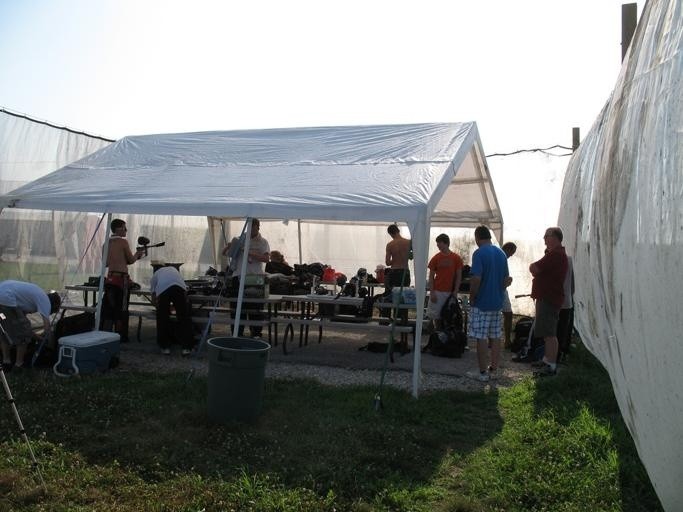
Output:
<box><xmin>108</xmin><ymin>273</ymin><xmax>129</xmax><ymax>277</ymax></box>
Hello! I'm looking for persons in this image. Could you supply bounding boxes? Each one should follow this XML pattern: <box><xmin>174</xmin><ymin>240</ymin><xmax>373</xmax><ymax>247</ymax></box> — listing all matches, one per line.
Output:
<box><xmin>384</xmin><ymin>224</ymin><xmax>411</xmax><ymax>317</ymax></box>
<box><xmin>425</xmin><ymin>234</ymin><xmax>463</xmax><ymax>329</ymax></box>
<box><xmin>221</xmin><ymin>219</ymin><xmax>270</xmax><ymax>338</ymax></box>
<box><xmin>101</xmin><ymin>218</ymin><xmax>142</xmax><ymax>343</ymax></box>
<box><xmin>529</xmin><ymin>227</ymin><xmax>568</xmax><ymax>377</ymax></box>
<box><xmin>469</xmin><ymin>226</ymin><xmax>509</xmax><ymax>381</ymax></box>
<box><xmin>151</xmin><ymin>265</ymin><xmax>192</xmax><ymax>355</ymax></box>
<box><xmin>488</xmin><ymin>243</ymin><xmax>516</xmax><ymax>349</ymax></box>
<box><xmin>265</xmin><ymin>251</ymin><xmax>293</xmax><ymax>274</ymax></box>
<box><xmin>511</xmin><ymin>249</ymin><xmax>574</xmax><ymax>364</ymax></box>
<box><xmin>0</xmin><ymin>280</ymin><xmax>60</xmax><ymax>368</ymax></box>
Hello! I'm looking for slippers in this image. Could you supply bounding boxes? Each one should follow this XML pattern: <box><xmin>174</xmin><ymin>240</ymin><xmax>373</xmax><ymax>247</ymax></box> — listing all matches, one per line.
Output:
<box><xmin>510</xmin><ymin>316</ymin><xmax>535</xmax><ymax>353</ymax></box>
<box><xmin>422</xmin><ymin>294</ymin><xmax>467</xmax><ymax>359</ymax></box>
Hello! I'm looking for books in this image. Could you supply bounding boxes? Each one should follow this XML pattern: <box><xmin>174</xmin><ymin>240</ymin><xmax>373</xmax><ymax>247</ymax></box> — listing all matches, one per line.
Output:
<box><xmin>58</xmin><ymin>331</ymin><xmax>121</xmax><ymax>374</ymax></box>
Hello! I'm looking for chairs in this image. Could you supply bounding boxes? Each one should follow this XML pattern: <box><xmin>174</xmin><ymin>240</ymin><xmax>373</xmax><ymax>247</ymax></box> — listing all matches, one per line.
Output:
<box><xmin>488</xmin><ymin>365</ymin><xmax>498</xmax><ymax>379</ymax></box>
<box><xmin>466</xmin><ymin>370</ymin><xmax>489</xmax><ymax>381</ymax></box>
<box><xmin>533</xmin><ymin>365</ymin><xmax>556</xmax><ymax>377</ymax></box>
<box><xmin>531</xmin><ymin>361</ymin><xmax>546</xmax><ymax>368</ymax></box>
<box><xmin>181</xmin><ymin>349</ymin><xmax>191</xmax><ymax>357</ymax></box>
<box><xmin>160</xmin><ymin>348</ymin><xmax>170</xmax><ymax>355</ymax></box>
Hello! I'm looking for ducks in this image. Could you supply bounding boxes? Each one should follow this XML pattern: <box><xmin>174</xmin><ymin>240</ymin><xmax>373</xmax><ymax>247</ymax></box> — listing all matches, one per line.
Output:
<box><xmin>271</xmin><ymin>280</ymin><xmax>311</xmax><ymax>295</ymax></box>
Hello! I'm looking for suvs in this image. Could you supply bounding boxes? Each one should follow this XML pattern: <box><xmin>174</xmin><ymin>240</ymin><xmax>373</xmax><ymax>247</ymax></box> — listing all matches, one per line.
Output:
<box><xmin>206</xmin><ymin>336</ymin><xmax>272</xmax><ymax>426</ymax></box>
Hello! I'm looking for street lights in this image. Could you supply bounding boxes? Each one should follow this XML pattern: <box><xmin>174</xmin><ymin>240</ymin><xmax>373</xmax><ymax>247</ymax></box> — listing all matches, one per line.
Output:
<box><xmin>358</xmin><ymin>339</ymin><xmax>411</xmax><ymax>358</ymax></box>
<box><xmin>83</xmin><ymin>277</ymin><xmax>106</xmax><ymax>286</ymax></box>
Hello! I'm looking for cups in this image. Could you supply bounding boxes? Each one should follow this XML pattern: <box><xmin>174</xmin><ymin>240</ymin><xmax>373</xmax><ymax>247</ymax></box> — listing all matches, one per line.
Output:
<box><xmin>544</xmin><ymin>235</ymin><xmax>553</xmax><ymax>239</ymax></box>
